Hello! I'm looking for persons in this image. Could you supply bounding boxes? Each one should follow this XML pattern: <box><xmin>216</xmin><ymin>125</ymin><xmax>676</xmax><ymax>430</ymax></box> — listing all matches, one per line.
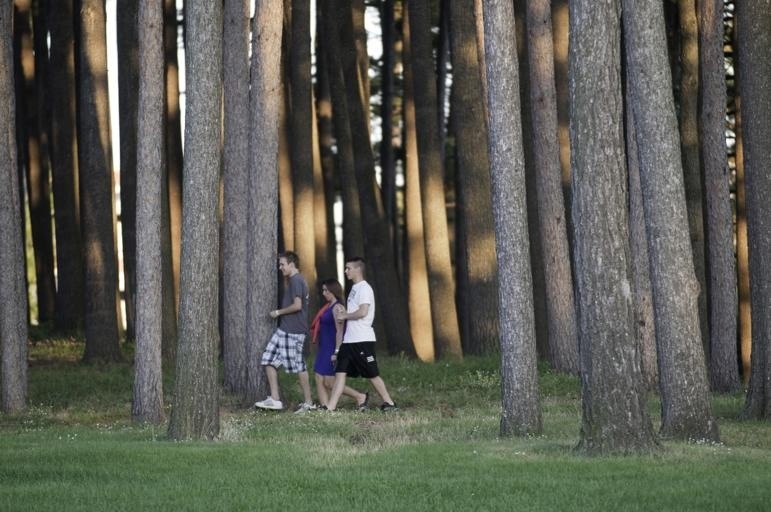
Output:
<box><xmin>254</xmin><ymin>249</ymin><xmax>318</xmax><ymax>417</ymax></box>
<box><xmin>309</xmin><ymin>278</ymin><xmax>370</xmax><ymax>411</ymax></box>
<box><xmin>318</xmin><ymin>258</ymin><xmax>398</xmax><ymax>411</ymax></box>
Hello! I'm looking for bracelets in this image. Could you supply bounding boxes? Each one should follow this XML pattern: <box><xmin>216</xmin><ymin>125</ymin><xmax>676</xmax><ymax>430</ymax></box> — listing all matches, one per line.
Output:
<box><xmin>275</xmin><ymin>310</ymin><xmax>281</xmax><ymax>317</ymax></box>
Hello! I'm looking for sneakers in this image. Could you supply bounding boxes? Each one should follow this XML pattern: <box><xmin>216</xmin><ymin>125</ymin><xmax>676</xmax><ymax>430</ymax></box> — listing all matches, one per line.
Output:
<box><xmin>254</xmin><ymin>396</ymin><xmax>283</xmax><ymax>410</ymax></box>
<box><xmin>380</xmin><ymin>402</ymin><xmax>398</xmax><ymax>412</ymax></box>
<box><xmin>294</xmin><ymin>402</ymin><xmax>336</xmax><ymax>414</ymax></box>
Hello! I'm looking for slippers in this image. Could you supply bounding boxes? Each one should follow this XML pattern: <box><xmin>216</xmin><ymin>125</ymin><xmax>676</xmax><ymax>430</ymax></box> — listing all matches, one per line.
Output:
<box><xmin>355</xmin><ymin>393</ymin><xmax>369</xmax><ymax>413</ymax></box>
